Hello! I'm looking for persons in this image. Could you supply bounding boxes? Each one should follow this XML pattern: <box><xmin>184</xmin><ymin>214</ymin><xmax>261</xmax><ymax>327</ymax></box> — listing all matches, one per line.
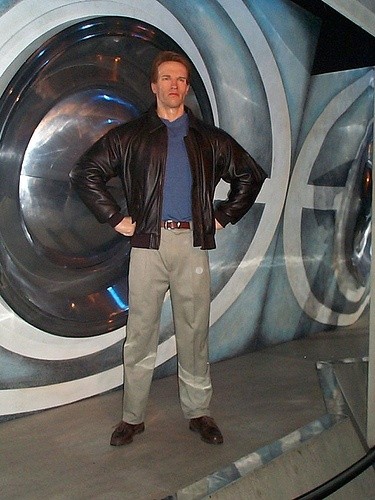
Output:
<box><xmin>71</xmin><ymin>49</ymin><xmax>267</xmax><ymax>448</ymax></box>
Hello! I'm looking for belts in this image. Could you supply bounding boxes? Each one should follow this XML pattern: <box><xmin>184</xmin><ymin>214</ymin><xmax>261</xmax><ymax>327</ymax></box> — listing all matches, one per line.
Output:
<box><xmin>161</xmin><ymin>218</ymin><xmax>190</xmax><ymax>231</ymax></box>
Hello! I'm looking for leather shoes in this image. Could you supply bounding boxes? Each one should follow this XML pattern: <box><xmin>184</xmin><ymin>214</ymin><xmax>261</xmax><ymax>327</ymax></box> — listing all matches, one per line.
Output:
<box><xmin>189</xmin><ymin>416</ymin><xmax>223</xmax><ymax>444</ymax></box>
<box><xmin>110</xmin><ymin>420</ymin><xmax>145</xmax><ymax>445</ymax></box>
